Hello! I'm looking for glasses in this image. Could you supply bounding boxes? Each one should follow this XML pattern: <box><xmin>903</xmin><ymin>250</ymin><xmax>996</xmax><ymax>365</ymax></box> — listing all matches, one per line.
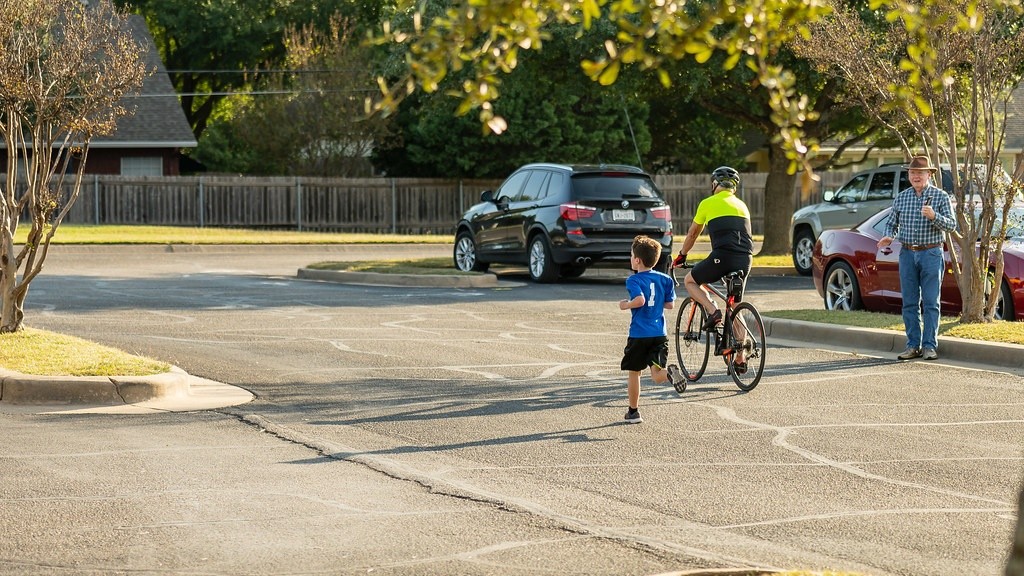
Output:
<box><xmin>907</xmin><ymin>170</ymin><xmax>928</xmax><ymax>176</ymax></box>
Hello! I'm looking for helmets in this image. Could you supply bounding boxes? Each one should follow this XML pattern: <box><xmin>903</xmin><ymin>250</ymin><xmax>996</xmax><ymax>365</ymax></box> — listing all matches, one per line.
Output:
<box><xmin>710</xmin><ymin>166</ymin><xmax>741</xmax><ymax>186</ymax></box>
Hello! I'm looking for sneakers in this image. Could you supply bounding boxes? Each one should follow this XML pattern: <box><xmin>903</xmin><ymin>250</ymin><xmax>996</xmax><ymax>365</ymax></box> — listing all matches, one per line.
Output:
<box><xmin>703</xmin><ymin>309</ymin><xmax>722</xmax><ymax>331</ymax></box>
<box><xmin>622</xmin><ymin>410</ymin><xmax>643</xmax><ymax>424</ymax></box>
<box><xmin>898</xmin><ymin>348</ymin><xmax>921</xmax><ymax>360</ymax></box>
<box><xmin>667</xmin><ymin>364</ymin><xmax>687</xmax><ymax>393</ymax></box>
<box><xmin>727</xmin><ymin>361</ymin><xmax>748</xmax><ymax>376</ymax></box>
<box><xmin>922</xmin><ymin>348</ymin><xmax>938</xmax><ymax>360</ymax></box>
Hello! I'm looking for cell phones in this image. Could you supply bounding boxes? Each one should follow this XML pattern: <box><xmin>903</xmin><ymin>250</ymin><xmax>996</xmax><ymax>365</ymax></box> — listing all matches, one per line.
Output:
<box><xmin>926</xmin><ymin>196</ymin><xmax>933</xmax><ymax>206</ymax></box>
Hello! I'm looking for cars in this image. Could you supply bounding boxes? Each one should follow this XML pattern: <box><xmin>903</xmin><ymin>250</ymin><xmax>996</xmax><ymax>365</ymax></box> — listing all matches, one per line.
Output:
<box><xmin>812</xmin><ymin>194</ymin><xmax>1024</xmax><ymax>323</ymax></box>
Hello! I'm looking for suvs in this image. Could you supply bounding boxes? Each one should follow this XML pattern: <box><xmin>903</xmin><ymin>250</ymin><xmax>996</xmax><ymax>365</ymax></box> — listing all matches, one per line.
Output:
<box><xmin>789</xmin><ymin>162</ymin><xmax>1024</xmax><ymax>277</ymax></box>
<box><xmin>453</xmin><ymin>161</ymin><xmax>673</xmax><ymax>284</ymax></box>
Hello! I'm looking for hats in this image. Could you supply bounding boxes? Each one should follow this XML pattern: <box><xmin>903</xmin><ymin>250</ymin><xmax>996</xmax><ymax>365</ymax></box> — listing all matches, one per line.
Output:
<box><xmin>903</xmin><ymin>156</ymin><xmax>938</xmax><ymax>173</ymax></box>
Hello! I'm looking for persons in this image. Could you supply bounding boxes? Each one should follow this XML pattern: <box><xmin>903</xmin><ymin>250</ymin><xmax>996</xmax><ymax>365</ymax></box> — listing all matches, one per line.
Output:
<box><xmin>673</xmin><ymin>166</ymin><xmax>755</xmax><ymax>376</ymax></box>
<box><xmin>619</xmin><ymin>235</ymin><xmax>688</xmax><ymax>425</ymax></box>
<box><xmin>876</xmin><ymin>156</ymin><xmax>957</xmax><ymax>361</ymax></box>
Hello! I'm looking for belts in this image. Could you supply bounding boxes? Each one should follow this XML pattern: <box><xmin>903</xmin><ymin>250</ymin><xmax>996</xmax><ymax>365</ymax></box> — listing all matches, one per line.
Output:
<box><xmin>902</xmin><ymin>242</ymin><xmax>941</xmax><ymax>251</ymax></box>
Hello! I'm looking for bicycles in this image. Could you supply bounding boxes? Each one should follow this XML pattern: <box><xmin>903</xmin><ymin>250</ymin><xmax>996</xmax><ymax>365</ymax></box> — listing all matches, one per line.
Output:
<box><xmin>671</xmin><ymin>263</ymin><xmax>766</xmax><ymax>392</ymax></box>
